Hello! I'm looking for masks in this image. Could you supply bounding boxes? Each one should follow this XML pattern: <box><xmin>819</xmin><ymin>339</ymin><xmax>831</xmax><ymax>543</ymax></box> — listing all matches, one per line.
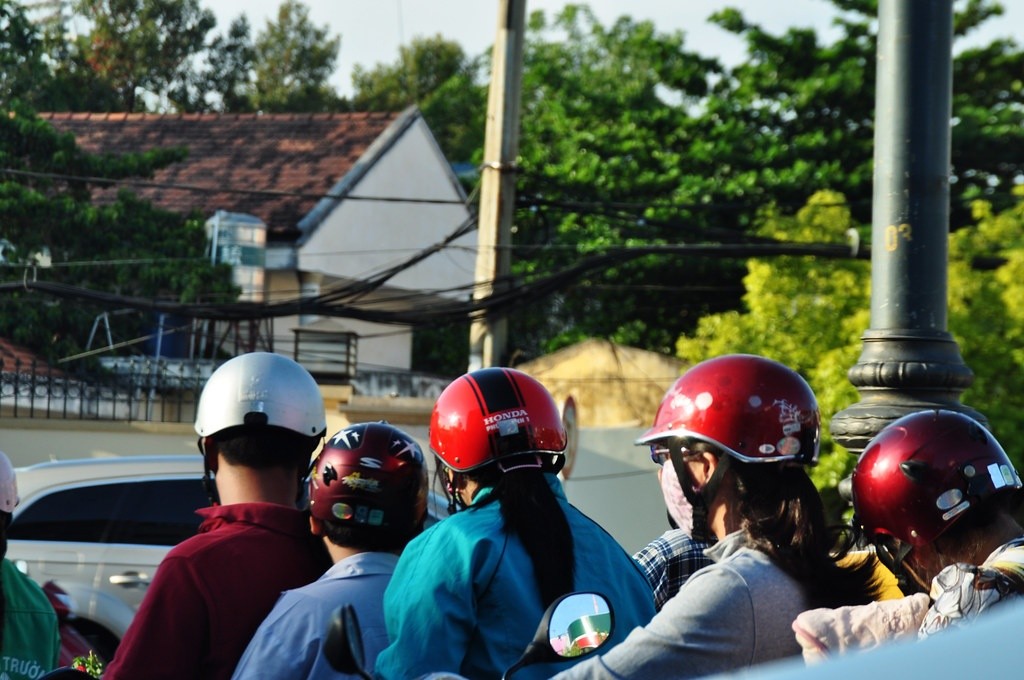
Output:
<box><xmin>658</xmin><ymin>458</ymin><xmax>700</xmax><ymax>541</ymax></box>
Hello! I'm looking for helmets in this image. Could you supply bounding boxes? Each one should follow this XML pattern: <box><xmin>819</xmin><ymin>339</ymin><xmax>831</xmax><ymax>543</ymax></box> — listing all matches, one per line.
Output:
<box><xmin>306</xmin><ymin>420</ymin><xmax>429</xmax><ymax>541</ymax></box>
<box><xmin>850</xmin><ymin>409</ymin><xmax>1023</xmax><ymax>548</ymax></box>
<box><xmin>0</xmin><ymin>451</ymin><xmax>18</xmax><ymax>514</ymax></box>
<box><xmin>634</xmin><ymin>353</ymin><xmax>822</xmax><ymax>467</ymax></box>
<box><xmin>193</xmin><ymin>351</ymin><xmax>328</xmax><ymax>437</ymax></box>
<box><xmin>426</xmin><ymin>367</ymin><xmax>569</xmax><ymax>473</ymax></box>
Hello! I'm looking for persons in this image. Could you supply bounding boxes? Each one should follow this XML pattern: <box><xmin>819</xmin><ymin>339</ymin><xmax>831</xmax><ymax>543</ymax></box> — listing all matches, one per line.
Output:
<box><xmin>0</xmin><ymin>450</ymin><xmax>60</xmax><ymax>680</ymax></box>
<box><xmin>633</xmin><ymin>526</ymin><xmax>717</xmax><ymax>615</ymax></box>
<box><xmin>229</xmin><ymin>421</ymin><xmax>428</xmax><ymax>680</ymax></box>
<box><xmin>373</xmin><ymin>367</ymin><xmax>658</xmax><ymax>680</ymax></box>
<box><xmin>852</xmin><ymin>409</ymin><xmax>1024</xmax><ymax>641</ymax></box>
<box><xmin>103</xmin><ymin>352</ymin><xmax>335</xmax><ymax>680</ymax></box>
<box><xmin>547</xmin><ymin>354</ymin><xmax>884</xmax><ymax>680</ymax></box>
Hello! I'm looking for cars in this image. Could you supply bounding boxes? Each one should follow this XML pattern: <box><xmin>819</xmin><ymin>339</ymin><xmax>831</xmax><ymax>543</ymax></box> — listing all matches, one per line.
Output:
<box><xmin>2</xmin><ymin>455</ymin><xmax>465</xmax><ymax>665</ymax></box>
